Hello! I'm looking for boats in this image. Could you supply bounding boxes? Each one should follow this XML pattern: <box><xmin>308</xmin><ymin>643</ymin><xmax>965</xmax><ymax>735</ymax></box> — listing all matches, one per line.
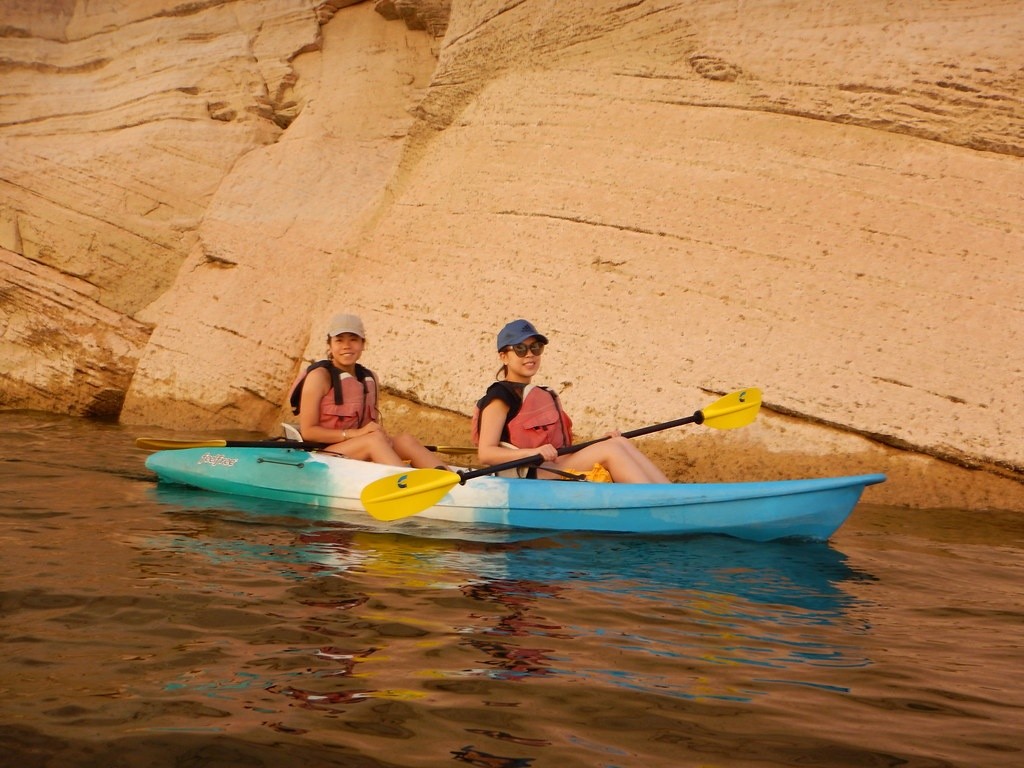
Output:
<box><xmin>142</xmin><ymin>422</ymin><xmax>889</xmax><ymax>547</ymax></box>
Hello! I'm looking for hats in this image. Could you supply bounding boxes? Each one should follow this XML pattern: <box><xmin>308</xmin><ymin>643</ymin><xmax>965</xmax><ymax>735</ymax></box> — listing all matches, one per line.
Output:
<box><xmin>326</xmin><ymin>313</ymin><xmax>366</xmax><ymax>338</ymax></box>
<box><xmin>496</xmin><ymin>318</ymin><xmax>549</xmax><ymax>352</ymax></box>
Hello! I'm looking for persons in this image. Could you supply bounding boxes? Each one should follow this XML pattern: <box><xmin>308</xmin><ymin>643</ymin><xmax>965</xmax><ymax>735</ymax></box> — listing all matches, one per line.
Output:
<box><xmin>299</xmin><ymin>314</ymin><xmax>455</xmax><ymax>472</ymax></box>
<box><xmin>477</xmin><ymin>319</ymin><xmax>674</xmax><ymax>485</ymax></box>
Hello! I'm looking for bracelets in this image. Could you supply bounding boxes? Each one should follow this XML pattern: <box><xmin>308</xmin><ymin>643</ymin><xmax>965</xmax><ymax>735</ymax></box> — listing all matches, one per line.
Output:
<box><xmin>342</xmin><ymin>428</ymin><xmax>347</xmax><ymax>441</ymax></box>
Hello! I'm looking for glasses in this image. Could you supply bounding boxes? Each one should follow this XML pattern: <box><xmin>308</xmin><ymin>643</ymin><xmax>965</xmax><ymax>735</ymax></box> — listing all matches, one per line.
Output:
<box><xmin>503</xmin><ymin>341</ymin><xmax>545</xmax><ymax>358</ymax></box>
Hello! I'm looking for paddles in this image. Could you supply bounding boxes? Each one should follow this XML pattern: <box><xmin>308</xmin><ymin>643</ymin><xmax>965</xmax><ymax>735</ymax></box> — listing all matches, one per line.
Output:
<box><xmin>523</xmin><ymin>463</ymin><xmax>587</xmax><ymax>481</ymax></box>
<box><xmin>361</xmin><ymin>387</ymin><xmax>763</xmax><ymax>522</ymax></box>
<box><xmin>133</xmin><ymin>437</ymin><xmax>479</xmax><ymax>456</ymax></box>
